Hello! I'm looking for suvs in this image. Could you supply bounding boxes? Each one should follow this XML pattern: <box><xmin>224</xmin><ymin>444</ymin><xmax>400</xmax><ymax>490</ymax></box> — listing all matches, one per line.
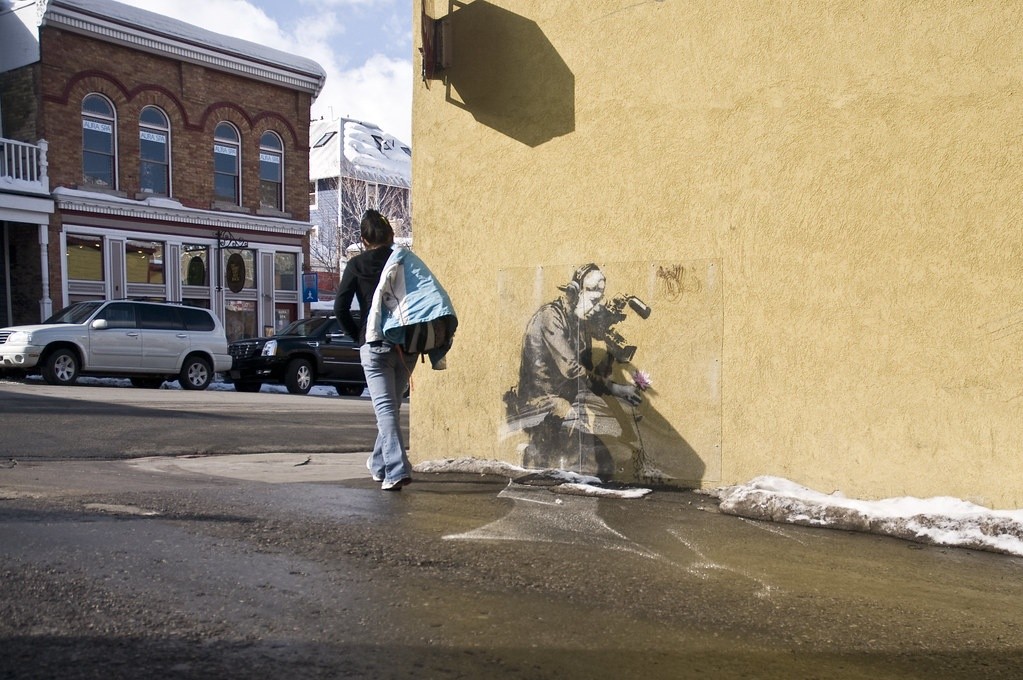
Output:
<box><xmin>228</xmin><ymin>312</ymin><xmax>411</xmax><ymax>397</ymax></box>
<box><xmin>0</xmin><ymin>299</ymin><xmax>234</xmax><ymax>391</ymax></box>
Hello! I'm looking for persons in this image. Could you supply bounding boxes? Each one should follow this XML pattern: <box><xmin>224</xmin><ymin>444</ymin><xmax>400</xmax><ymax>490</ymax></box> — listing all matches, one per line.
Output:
<box><xmin>333</xmin><ymin>208</ymin><xmax>421</xmax><ymax>492</ymax></box>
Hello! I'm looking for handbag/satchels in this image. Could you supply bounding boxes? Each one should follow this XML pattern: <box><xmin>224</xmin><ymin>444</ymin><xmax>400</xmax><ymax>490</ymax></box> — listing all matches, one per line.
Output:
<box><xmin>396</xmin><ymin>317</ymin><xmax>446</xmax><ymax>354</ymax></box>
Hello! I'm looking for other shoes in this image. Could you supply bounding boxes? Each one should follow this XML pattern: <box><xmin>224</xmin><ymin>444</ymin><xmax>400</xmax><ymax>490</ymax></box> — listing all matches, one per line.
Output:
<box><xmin>381</xmin><ymin>474</ymin><xmax>411</xmax><ymax>490</ymax></box>
<box><xmin>366</xmin><ymin>457</ymin><xmax>383</xmax><ymax>481</ymax></box>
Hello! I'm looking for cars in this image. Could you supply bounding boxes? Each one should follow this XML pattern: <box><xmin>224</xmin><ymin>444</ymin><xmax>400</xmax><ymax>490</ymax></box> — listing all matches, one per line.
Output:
<box><xmin>149</xmin><ymin>255</ymin><xmax>164</xmax><ymax>270</ymax></box>
